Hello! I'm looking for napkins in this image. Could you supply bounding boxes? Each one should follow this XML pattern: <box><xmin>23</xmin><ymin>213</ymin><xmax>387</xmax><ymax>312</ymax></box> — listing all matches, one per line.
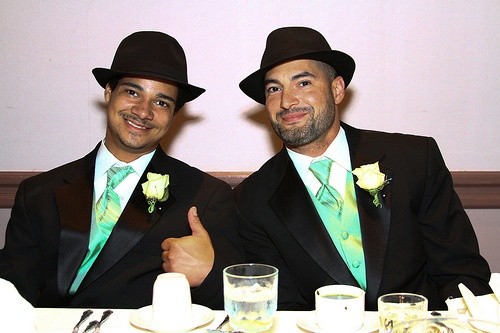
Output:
<box><xmin>446</xmin><ymin>272</ymin><xmax>500</xmax><ymax>333</ymax></box>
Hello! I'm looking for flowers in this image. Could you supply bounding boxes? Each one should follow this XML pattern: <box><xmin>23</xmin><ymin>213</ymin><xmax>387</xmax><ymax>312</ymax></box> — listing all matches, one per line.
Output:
<box><xmin>352</xmin><ymin>161</ymin><xmax>393</xmax><ymax>208</ymax></box>
<box><xmin>140</xmin><ymin>172</ymin><xmax>171</xmax><ymax>214</ymax></box>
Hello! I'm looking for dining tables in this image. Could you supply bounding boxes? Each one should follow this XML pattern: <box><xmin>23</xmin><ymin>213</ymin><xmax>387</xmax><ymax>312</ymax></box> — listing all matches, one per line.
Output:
<box><xmin>34</xmin><ymin>307</ymin><xmax>476</xmax><ymax>333</ymax></box>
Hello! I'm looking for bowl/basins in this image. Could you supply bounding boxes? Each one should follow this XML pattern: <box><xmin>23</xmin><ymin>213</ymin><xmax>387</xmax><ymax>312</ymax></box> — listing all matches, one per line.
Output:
<box><xmin>379</xmin><ymin>316</ymin><xmax>500</xmax><ymax>333</ymax></box>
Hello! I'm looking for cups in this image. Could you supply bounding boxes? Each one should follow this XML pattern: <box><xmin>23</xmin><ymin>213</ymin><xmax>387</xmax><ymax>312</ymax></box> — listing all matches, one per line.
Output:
<box><xmin>378</xmin><ymin>292</ymin><xmax>428</xmax><ymax>333</ymax></box>
<box><xmin>315</xmin><ymin>284</ymin><xmax>365</xmax><ymax>332</ymax></box>
<box><xmin>152</xmin><ymin>272</ymin><xmax>192</xmax><ymax>325</ymax></box>
<box><xmin>222</xmin><ymin>263</ymin><xmax>279</xmax><ymax>333</ymax></box>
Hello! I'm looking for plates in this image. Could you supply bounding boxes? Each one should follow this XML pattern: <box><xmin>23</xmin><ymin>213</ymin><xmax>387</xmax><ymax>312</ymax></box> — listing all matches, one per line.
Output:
<box><xmin>296</xmin><ymin>310</ymin><xmax>379</xmax><ymax>333</ymax></box>
<box><xmin>128</xmin><ymin>303</ymin><xmax>215</xmax><ymax>333</ymax></box>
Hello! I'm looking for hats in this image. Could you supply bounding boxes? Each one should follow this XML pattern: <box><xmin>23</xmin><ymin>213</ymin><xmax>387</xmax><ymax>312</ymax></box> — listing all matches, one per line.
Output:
<box><xmin>239</xmin><ymin>26</ymin><xmax>356</xmax><ymax>105</ymax></box>
<box><xmin>92</xmin><ymin>31</ymin><xmax>206</xmax><ymax>102</ymax></box>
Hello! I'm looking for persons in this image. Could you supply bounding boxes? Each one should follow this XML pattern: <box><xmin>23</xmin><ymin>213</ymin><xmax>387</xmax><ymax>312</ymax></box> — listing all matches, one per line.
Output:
<box><xmin>228</xmin><ymin>27</ymin><xmax>495</xmax><ymax>312</ymax></box>
<box><xmin>0</xmin><ymin>31</ymin><xmax>242</xmax><ymax>318</ymax></box>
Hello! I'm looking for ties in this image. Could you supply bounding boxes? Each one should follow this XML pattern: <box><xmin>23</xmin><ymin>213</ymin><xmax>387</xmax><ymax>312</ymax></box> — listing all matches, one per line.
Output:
<box><xmin>309</xmin><ymin>159</ymin><xmax>344</xmax><ymax>222</ymax></box>
<box><xmin>95</xmin><ymin>166</ymin><xmax>135</xmax><ymax>240</ymax></box>
